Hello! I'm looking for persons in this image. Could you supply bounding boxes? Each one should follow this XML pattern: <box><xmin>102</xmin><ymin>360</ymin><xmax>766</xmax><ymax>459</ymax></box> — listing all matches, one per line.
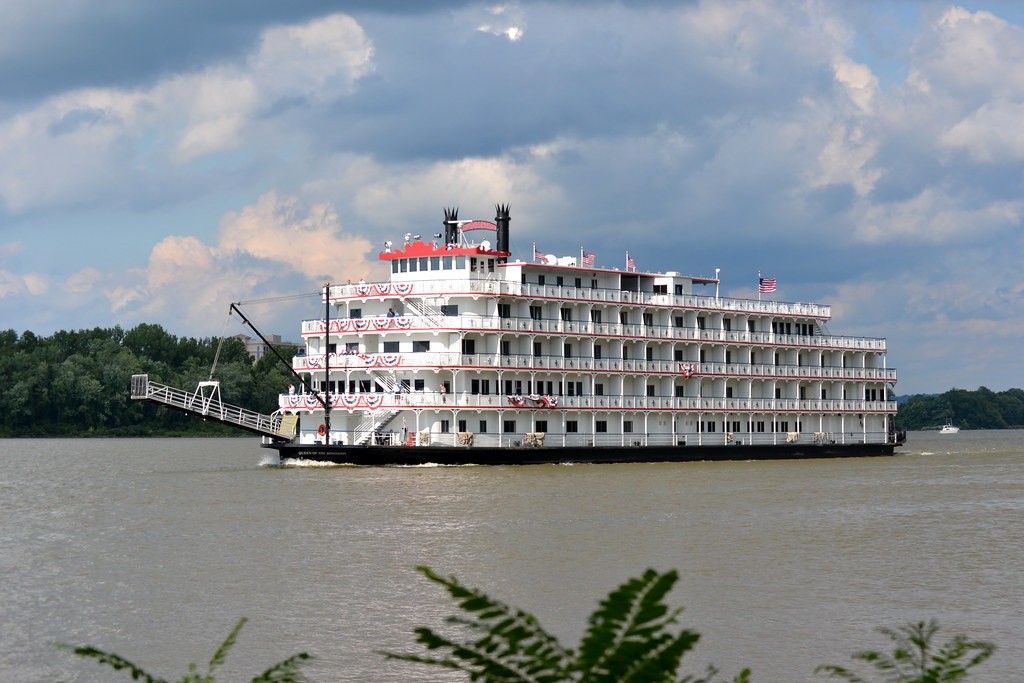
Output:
<box><xmin>346</xmin><ymin>344</ymin><xmax>354</xmax><ymax>355</ymax></box>
<box><xmin>439</xmin><ymin>383</ymin><xmax>447</xmax><ymax>394</ymax></box>
<box><xmin>394</xmin><ymin>309</ymin><xmax>401</xmax><ymax>317</ymax></box>
<box><xmin>387</xmin><ymin>307</ymin><xmax>395</xmax><ymax>317</ymax></box>
<box><xmin>288</xmin><ymin>383</ymin><xmax>295</xmax><ymax>395</ymax></box>
<box><xmin>393</xmin><ymin>377</ymin><xmax>404</xmax><ymax>405</ymax></box>
<box><xmin>479</xmin><ymin>235</ymin><xmax>492</xmax><ymax>252</ymax></box>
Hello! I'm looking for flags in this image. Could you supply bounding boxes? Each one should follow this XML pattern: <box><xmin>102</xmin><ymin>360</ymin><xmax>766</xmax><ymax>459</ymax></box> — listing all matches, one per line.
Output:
<box><xmin>583</xmin><ymin>247</ymin><xmax>595</xmax><ymax>265</ymax></box>
<box><xmin>628</xmin><ymin>257</ymin><xmax>636</xmax><ymax>269</ymax></box>
<box><xmin>535</xmin><ymin>249</ymin><xmax>549</xmax><ymax>264</ymax></box>
<box><xmin>758</xmin><ymin>277</ymin><xmax>777</xmax><ymax>294</ymax></box>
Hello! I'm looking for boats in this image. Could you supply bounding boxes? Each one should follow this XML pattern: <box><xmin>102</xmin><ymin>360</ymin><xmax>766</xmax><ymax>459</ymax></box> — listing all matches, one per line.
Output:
<box><xmin>258</xmin><ymin>204</ymin><xmax>911</xmax><ymax>465</ymax></box>
<box><xmin>938</xmin><ymin>417</ymin><xmax>961</xmax><ymax>434</ymax></box>
